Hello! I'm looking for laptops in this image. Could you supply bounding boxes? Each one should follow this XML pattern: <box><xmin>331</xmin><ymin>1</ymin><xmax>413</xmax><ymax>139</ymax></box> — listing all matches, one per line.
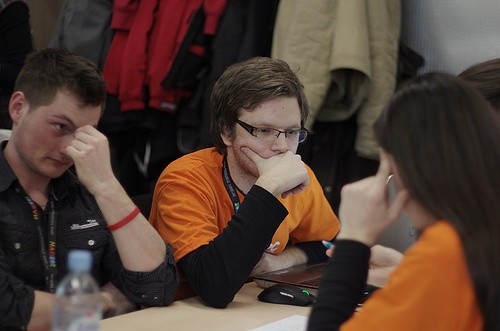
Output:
<box><xmin>252</xmin><ymin>258</ymin><xmax>326</xmax><ymax>291</ymax></box>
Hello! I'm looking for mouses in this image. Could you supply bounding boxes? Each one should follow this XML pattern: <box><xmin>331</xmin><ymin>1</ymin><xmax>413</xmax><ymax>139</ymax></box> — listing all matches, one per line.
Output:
<box><xmin>256</xmin><ymin>283</ymin><xmax>317</xmax><ymax>306</ymax></box>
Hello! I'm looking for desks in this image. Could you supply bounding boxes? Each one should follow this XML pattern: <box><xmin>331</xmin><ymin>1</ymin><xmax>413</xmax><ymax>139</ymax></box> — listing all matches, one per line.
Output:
<box><xmin>101</xmin><ymin>280</ymin><xmax>313</xmax><ymax>331</ymax></box>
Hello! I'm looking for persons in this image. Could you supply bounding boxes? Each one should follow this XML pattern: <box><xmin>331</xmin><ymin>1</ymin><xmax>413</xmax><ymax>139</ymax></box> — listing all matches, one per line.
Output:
<box><xmin>304</xmin><ymin>70</ymin><xmax>500</xmax><ymax>331</ymax></box>
<box><xmin>326</xmin><ymin>58</ymin><xmax>500</xmax><ymax>287</ymax></box>
<box><xmin>149</xmin><ymin>56</ymin><xmax>341</xmax><ymax>309</ymax></box>
<box><xmin>0</xmin><ymin>48</ymin><xmax>180</xmax><ymax>331</ymax></box>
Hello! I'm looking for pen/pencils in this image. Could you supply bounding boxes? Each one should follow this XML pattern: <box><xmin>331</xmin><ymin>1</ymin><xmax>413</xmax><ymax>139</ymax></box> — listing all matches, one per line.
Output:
<box><xmin>322</xmin><ymin>241</ymin><xmax>334</xmax><ymax>249</ymax></box>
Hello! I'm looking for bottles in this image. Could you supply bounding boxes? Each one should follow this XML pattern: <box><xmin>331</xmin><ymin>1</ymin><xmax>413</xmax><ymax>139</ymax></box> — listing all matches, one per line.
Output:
<box><xmin>52</xmin><ymin>250</ymin><xmax>102</xmax><ymax>331</ymax></box>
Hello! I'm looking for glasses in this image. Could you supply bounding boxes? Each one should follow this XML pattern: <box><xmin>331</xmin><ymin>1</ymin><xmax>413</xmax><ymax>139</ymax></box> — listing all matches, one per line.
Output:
<box><xmin>233</xmin><ymin>120</ymin><xmax>307</xmax><ymax>144</ymax></box>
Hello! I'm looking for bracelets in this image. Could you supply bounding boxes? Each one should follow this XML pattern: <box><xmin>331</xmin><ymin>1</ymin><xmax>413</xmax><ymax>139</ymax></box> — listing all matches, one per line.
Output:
<box><xmin>107</xmin><ymin>207</ymin><xmax>140</xmax><ymax>231</ymax></box>
